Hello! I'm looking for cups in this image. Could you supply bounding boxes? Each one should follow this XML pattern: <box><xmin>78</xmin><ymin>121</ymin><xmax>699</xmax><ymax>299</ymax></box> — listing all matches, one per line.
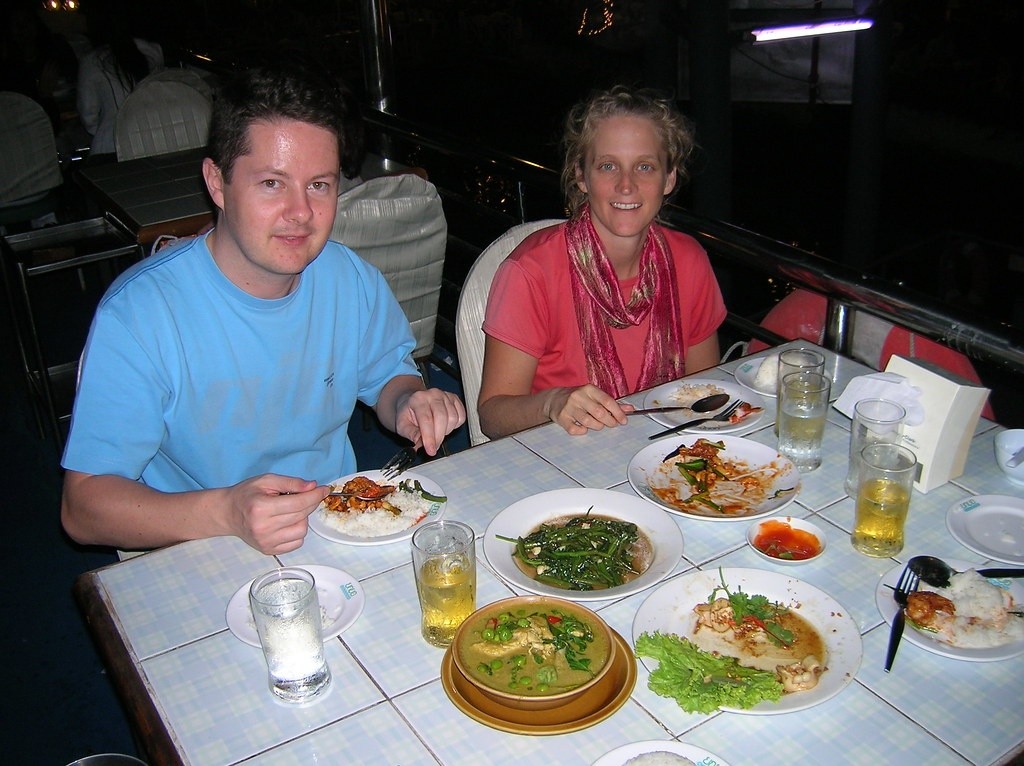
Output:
<box><xmin>843</xmin><ymin>396</ymin><xmax>906</xmax><ymax>498</ymax></box>
<box><xmin>249</xmin><ymin>567</ymin><xmax>332</xmax><ymax>705</ymax></box>
<box><xmin>850</xmin><ymin>442</ymin><xmax>917</xmax><ymax>558</ymax></box>
<box><xmin>411</xmin><ymin>520</ymin><xmax>476</xmax><ymax>649</ymax></box>
<box><xmin>774</xmin><ymin>372</ymin><xmax>831</xmax><ymax>472</ymax></box>
<box><xmin>773</xmin><ymin>348</ymin><xmax>825</xmax><ymax>437</ymax></box>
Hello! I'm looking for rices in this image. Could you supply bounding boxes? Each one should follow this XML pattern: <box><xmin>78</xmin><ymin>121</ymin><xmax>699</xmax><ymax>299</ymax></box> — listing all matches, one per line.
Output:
<box><xmin>669</xmin><ymin>384</ymin><xmax>726</xmax><ymax>399</ymax></box>
<box><xmin>318</xmin><ymin>479</ymin><xmax>431</xmax><ymax>537</ymax></box>
<box><xmin>935</xmin><ymin>567</ymin><xmax>1024</xmax><ymax>647</ymax></box>
<box><xmin>753</xmin><ymin>352</ymin><xmax>809</xmax><ymax>387</ymax></box>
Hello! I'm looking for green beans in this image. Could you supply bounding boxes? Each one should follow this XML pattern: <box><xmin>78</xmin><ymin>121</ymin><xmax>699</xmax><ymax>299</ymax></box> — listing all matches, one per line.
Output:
<box><xmin>399</xmin><ymin>479</ymin><xmax>448</xmax><ymax>502</ymax></box>
<box><xmin>477</xmin><ymin>611</ymin><xmax>549</xmax><ymax>693</ymax></box>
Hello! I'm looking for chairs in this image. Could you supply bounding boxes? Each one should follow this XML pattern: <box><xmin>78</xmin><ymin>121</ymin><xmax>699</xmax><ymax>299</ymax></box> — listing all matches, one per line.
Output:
<box><xmin>113</xmin><ymin>81</ymin><xmax>214</xmax><ymax>162</ymax></box>
<box><xmin>328</xmin><ymin>174</ymin><xmax>447</xmax><ymax>386</ymax></box>
<box><xmin>455</xmin><ymin>219</ymin><xmax>569</xmax><ymax>448</ymax></box>
<box><xmin>0</xmin><ymin>91</ymin><xmax>72</xmax><ymax>218</ymax></box>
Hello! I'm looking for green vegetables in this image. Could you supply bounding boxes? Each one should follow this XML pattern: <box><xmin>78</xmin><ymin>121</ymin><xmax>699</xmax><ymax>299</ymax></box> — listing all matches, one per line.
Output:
<box><xmin>496</xmin><ymin>506</ymin><xmax>641</xmax><ymax>590</ymax></box>
<box><xmin>675</xmin><ymin>458</ymin><xmax>730</xmax><ymax>514</ymax></box>
<box><xmin>708</xmin><ymin>566</ymin><xmax>794</xmax><ymax>647</ymax></box>
<box><xmin>527</xmin><ymin>609</ymin><xmax>596</xmax><ymax>679</ymax></box>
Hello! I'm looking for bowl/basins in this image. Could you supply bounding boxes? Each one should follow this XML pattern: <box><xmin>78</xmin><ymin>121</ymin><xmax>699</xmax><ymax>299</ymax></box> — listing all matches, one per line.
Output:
<box><xmin>994</xmin><ymin>429</ymin><xmax>1024</xmax><ymax>486</ymax></box>
<box><xmin>450</xmin><ymin>595</ymin><xmax>616</xmax><ymax>711</ymax></box>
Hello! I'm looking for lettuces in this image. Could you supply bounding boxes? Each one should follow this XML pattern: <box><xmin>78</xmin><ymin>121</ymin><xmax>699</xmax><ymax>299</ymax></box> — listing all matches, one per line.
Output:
<box><xmin>635</xmin><ymin>629</ymin><xmax>783</xmax><ymax>714</ymax></box>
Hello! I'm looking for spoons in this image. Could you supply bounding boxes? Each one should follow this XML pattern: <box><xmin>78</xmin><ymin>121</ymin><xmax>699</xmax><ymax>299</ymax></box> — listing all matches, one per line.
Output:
<box><xmin>288</xmin><ymin>486</ymin><xmax>395</xmax><ymax>500</ymax></box>
<box><xmin>623</xmin><ymin>394</ymin><xmax>730</xmax><ymax>416</ymax></box>
<box><xmin>908</xmin><ymin>556</ymin><xmax>1024</xmax><ymax>588</ymax></box>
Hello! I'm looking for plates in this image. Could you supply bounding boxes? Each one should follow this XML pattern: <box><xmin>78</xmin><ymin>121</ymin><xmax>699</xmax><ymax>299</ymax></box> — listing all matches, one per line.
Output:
<box><xmin>483</xmin><ymin>487</ymin><xmax>684</xmax><ymax>601</ymax></box>
<box><xmin>441</xmin><ymin>626</ymin><xmax>637</xmax><ymax>736</ymax></box>
<box><xmin>945</xmin><ymin>495</ymin><xmax>1024</xmax><ymax>566</ymax></box>
<box><xmin>644</xmin><ymin>379</ymin><xmax>766</xmax><ymax>433</ymax></box>
<box><xmin>734</xmin><ymin>357</ymin><xmax>832</xmax><ymax>398</ymax></box>
<box><xmin>225</xmin><ymin>564</ymin><xmax>366</xmax><ymax>650</ymax></box>
<box><xmin>308</xmin><ymin>469</ymin><xmax>447</xmax><ymax>547</ymax></box>
<box><xmin>627</xmin><ymin>434</ymin><xmax>802</xmax><ymax>521</ymax></box>
<box><xmin>591</xmin><ymin>740</ymin><xmax>731</xmax><ymax>766</ymax></box>
<box><xmin>875</xmin><ymin>558</ymin><xmax>1024</xmax><ymax>662</ymax></box>
<box><xmin>746</xmin><ymin>516</ymin><xmax>828</xmax><ymax>567</ymax></box>
<box><xmin>632</xmin><ymin>567</ymin><xmax>863</xmax><ymax>716</ymax></box>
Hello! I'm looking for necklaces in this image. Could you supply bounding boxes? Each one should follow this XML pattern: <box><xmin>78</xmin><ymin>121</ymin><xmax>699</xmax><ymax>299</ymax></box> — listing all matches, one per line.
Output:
<box><xmin>284</xmin><ymin>274</ymin><xmax>296</xmax><ymax>299</ymax></box>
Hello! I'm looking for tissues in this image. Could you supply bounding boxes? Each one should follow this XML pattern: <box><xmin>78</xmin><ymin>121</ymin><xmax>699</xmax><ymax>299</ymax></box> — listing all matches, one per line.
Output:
<box><xmin>829</xmin><ymin>352</ymin><xmax>991</xmax><ymax>494</ymax></box>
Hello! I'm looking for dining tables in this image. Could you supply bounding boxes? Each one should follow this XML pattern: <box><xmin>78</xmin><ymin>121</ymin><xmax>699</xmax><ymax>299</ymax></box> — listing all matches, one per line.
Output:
<box><xmin>77</xmin><ymin>337</ymin><xmax>1023</xmax><ymax>765</ymax></box>
<box><xmin>74</xmin><ymin>147</ymin><xmax>219</xmax><ymax>246</ymax></box>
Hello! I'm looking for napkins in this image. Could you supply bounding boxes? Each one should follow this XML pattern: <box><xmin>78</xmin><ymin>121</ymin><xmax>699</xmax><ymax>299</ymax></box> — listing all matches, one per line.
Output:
<box><xmin>834</xmin><ymin>374</ymin><xmax>922</xmax><ymax>425</ymax></box>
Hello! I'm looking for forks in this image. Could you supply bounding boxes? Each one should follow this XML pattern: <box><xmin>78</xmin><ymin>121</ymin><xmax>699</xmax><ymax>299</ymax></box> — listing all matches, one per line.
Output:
<box><xmin>380</xmin><ymin>436</ymin><xmax>423</xmax><ymax>481</ymax></box>
<box><xmin>648</xmin><ymin>399</ymin><xmax>745</xmax><ymax>440</ymax></box>
<box><xmin>884</xmin><ymin>564</ymin><xmax>922</xmax><ymax>673</ymax></box>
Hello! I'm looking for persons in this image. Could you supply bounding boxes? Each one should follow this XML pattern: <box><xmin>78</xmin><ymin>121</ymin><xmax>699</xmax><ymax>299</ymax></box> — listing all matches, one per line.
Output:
<box><xmin>0</xmin><ymin>0</ymin><xmax>168</xmax><ymax>156</ymax></box>
<box><xmin>476</xmin><ymin>84</ymin><xmax>727</xmax><ymax>440</ymax></box>
<box><xmin>60</xmin><ymin>62</ymin><xmax>466</xmax><ymax>555</ymax></box>
